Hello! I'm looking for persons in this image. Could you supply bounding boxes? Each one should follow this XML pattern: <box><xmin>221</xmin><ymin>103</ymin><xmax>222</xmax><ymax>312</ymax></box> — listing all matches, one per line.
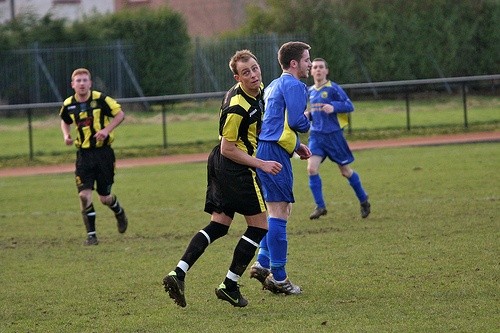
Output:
<box><xmin>248</xmin><ymin>42</ymin><xmax>312</xmax><ymax>294</ymax></box>
<box><xmin>59</xmin><ymin>69</ymin><xmax>128</xmax><ymax>246</ymax></box>
<box><xmin>306</xmin><ymin>58</ymin><xmax>370</xmax><ymax>220</ymax></box>
<box><xmin>162</xmin><ymin>49</ymin><xmax>283</xmax><ymax>308</ymax></box>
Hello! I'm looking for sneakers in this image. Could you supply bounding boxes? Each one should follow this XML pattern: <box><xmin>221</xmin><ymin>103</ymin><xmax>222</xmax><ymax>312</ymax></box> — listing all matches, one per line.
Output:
<box><xmin>250</xmin><ymin>262</ymin><xmax>270</xmax><ymax>283</ymax></box>
<box><xmin>162</xmin><ymin>270</ymin><xmax>187</xmax><ymax>307</ymax></box>
<box><xmin>310</xmin><ymin>207</ymin><xmax>327</xmax><ymax>219</ymax></box>
<box><xmin>216</xmin><ymin>282</ymin><xmax>248</xmax><ymax>307</ymax></box>
<box><xmin>115</xmin><ymin>208</ymin><xmax>126</xmax><ymax>233</ymax></box>
<box><xmin>264</xmin><ymin>272</ymin><xmax>301</xmax><ymax>296</ymax></box>
<box><xmin>360</xmin><ymin>201</ymin><xmax>371</xmax><ymax>218</ymax></box>
<box><xmin>85</xmin><ymin>237</ymin><xmax>97</xmax><ymax>245</ymax></box>
